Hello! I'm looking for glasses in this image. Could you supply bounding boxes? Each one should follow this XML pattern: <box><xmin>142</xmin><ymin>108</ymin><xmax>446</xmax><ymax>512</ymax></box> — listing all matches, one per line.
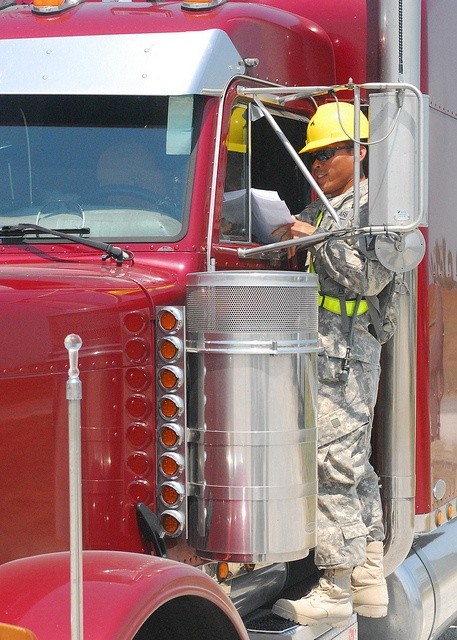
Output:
<box><xmin>307</xmin><ymin>146</ymin><xmax>352</xmax><ymax>163</ymax></box>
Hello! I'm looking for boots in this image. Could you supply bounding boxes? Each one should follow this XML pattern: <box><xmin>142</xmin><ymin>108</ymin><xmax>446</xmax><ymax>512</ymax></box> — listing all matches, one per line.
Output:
<box><xmin>273</xmin><ymin>569</ymin><xmax>352</xmax><ymax>626</ymax></box>
<box><xmin>351</xmin><ymin>541</ymin><xmax>388</xmax><ymax>618</ymax></box>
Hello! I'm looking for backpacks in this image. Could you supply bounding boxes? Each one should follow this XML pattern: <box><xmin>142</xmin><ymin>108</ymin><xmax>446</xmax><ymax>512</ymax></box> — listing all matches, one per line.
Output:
<box><xmin>380</xmin><ymin>283</ymin><xmax>399</xmax><ymax>345</ymax></box>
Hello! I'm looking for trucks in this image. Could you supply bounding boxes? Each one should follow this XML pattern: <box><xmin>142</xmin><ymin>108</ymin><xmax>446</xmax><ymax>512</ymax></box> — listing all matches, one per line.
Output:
<box><xmin>0</xmin><ymin>0</ymin><xmax>457</xmax><ymax>640</ymax></box>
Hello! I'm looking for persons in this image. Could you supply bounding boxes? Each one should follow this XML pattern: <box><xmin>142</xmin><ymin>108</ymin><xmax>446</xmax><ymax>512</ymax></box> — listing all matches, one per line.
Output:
<box><xmin>270</xmin><ymin>101</ymin><xmax>397</xmax><ymax>624</ymax></box>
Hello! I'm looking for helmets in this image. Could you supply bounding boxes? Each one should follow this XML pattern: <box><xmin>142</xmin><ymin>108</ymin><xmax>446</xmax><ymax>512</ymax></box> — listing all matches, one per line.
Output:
<box><xmin>297</xmin><ymin>102</ymin><xmax>369</xmax><ymax>154</ymax></box>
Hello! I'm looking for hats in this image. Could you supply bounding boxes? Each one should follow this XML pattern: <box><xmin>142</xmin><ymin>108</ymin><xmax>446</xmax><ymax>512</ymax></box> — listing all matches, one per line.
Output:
<box><xmin>227</xmin><ymin>107</ymin><xmax>247</xmax><ymax>154</ymax></box>
